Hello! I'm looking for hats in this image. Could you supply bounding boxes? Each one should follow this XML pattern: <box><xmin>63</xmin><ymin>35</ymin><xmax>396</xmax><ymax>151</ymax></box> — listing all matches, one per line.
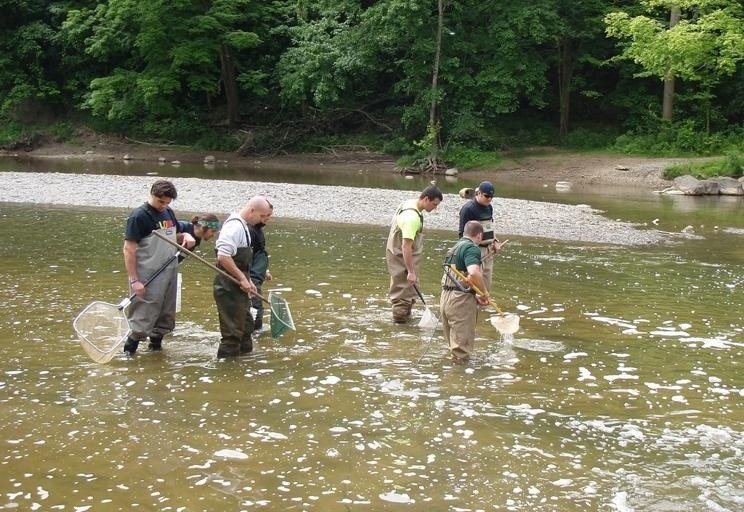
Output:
<box><xmin>475</xmin><ymin>181</ymin><xmax>494</xmax><ymax>194</ymax></box>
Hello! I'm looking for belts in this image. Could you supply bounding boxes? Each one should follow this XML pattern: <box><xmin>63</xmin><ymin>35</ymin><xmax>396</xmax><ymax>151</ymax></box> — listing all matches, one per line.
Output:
<box><xmin>443</xmin><ymin>285</ymin><xmax>476</xmax><ymax>294</ymax></box>
<box><xmin>478</xmin><ymin>244</ymin><xmax>489</xmax><ymax>248</ymax></box>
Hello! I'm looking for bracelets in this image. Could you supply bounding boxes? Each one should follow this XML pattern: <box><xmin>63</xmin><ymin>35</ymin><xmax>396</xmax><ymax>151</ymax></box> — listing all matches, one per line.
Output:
<box><xmin>494</xmin><ymin>239</ymin><xmax>499</xmax><ymax>242</ymax></box>
<box><xmin>131</xmin><ymin>279</ymin><xmax>138</xmax><ymax>284</ymax></box>
<box><xmin>266</xmin><ymin>269</ymin><xmax>269</xmax><ymax>273</ymax></box>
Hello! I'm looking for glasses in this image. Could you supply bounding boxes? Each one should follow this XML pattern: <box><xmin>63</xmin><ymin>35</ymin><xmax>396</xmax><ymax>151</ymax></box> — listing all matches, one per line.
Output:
<box><xmin>484</xmin><ymin>194</ymin><xmax>493</xmax><ymax>198</ymax></box>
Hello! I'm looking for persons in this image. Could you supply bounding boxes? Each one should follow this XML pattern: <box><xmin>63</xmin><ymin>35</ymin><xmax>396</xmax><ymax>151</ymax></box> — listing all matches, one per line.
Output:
<box><xmin>386</xmin><ymin>184</ymin><xmax>442</xmax><ymax>323</ymax></box>
<box><xmin>213</xmin><ymin>196</ymin><xmax>270</xmax><ymax>359</ymax></box>
<box><xmin>123</xmin><ymin>181</ymin><xmax>196</xmax><ymax>355</ymax></box>
<box><xmin>440</xmin><ymin>220</ymin><xmax>490</xmax><ymax>361</ymax></box>
<box><xmin>459</xmin><ymin>181</ymin><xmax>501</xmax><ymax>293</ymax></box>
<box><xmin>247</xmin><ymin>200</ymin><xmax>272</xmax><ymax>331</ymax></box>
<box><xmin>176</xmin><ymin>213</ymin><xmax>220</xmax><ymax>263</ymax></box>
